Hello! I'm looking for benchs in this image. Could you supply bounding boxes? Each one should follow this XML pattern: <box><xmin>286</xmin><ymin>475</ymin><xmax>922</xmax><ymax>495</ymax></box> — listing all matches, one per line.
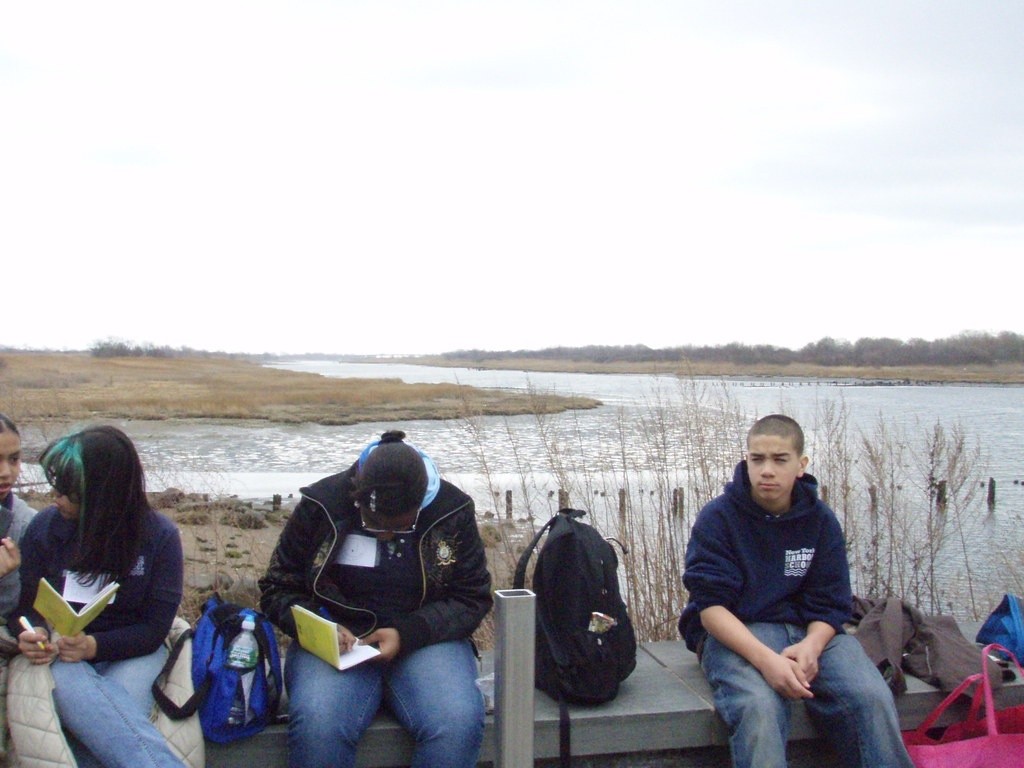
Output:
<box><xmin>639</xmin><ymin>621</ymin><xmax>1024</xmax><ymax>747</ymax></box>
<box><xmin>204</xmin><ymin>640</ymin><xmax>711</xmax><ymax>768</ymax></box>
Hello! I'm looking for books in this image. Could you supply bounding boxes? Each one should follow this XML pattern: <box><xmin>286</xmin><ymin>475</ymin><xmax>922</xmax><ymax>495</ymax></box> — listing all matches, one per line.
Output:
<box><xmin>290</xmin><ymin>603</ymin><xmax>382</xmax><ymax>670</ymax></box>
<box><xmin>33</xmin><ymin>578</ymin><xmax>120</xmax><ymax>637</ymax></box>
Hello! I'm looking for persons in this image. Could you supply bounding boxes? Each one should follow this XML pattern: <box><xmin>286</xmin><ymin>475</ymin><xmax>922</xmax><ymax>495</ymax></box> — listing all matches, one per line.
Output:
<box><xmin>1</xmin><ymin>412</ymin><xmax>186</xmax><ymax>768</ymax></box>
<box><xmin>257</xmin><ymin>432</ymin><xmax>494</xmax><ymax>768</ymax></box>
<box><xmin>679</xmin><ymin>414</ymin><xmax>913</xmax><ymax>768</ymax></box>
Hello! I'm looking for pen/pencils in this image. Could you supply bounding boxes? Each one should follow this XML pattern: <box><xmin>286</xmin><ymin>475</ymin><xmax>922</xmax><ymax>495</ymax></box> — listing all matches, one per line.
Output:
<box><xmin>19</xmin><ymin>616</ymin><xmax>45</xmax><ymax>650</ymax></box>
<box><xmin>320</xmin><ymin>608</ymin><xmax>333</xmax><ymax>623</ymax></box>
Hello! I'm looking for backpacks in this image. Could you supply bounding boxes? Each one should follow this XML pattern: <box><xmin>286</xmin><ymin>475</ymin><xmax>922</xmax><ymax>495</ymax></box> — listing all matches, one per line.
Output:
<box><xmin>512</xmin><ymin>508</ymin><xmax>637</xmax><ymax>708</ymax></box>
<box><xmin>152</xmin><ymin>591</ymin><xmax>283</xmax><ymax>746</ymax></box>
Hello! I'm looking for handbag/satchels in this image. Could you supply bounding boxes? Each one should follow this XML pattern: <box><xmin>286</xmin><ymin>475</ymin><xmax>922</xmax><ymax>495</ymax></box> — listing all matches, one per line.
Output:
<box><xmin>902</xmin><ymin>642</ymin><xmax>1024</xmax><ymax>768</ymax></box>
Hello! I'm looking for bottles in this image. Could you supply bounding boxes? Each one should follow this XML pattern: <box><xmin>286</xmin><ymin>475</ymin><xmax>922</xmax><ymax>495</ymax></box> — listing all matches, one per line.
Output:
<box><xmin>223</xmin><ymin>614</ymin><xmax>259</xmax><ymax>727</ymax></box>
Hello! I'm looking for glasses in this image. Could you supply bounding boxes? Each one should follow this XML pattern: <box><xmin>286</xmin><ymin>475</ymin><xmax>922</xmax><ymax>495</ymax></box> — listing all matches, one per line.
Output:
<box><xmin>44</xmin><ymin>468</ymin><xmax>81</xmax><ymax>504</ymax></box>
